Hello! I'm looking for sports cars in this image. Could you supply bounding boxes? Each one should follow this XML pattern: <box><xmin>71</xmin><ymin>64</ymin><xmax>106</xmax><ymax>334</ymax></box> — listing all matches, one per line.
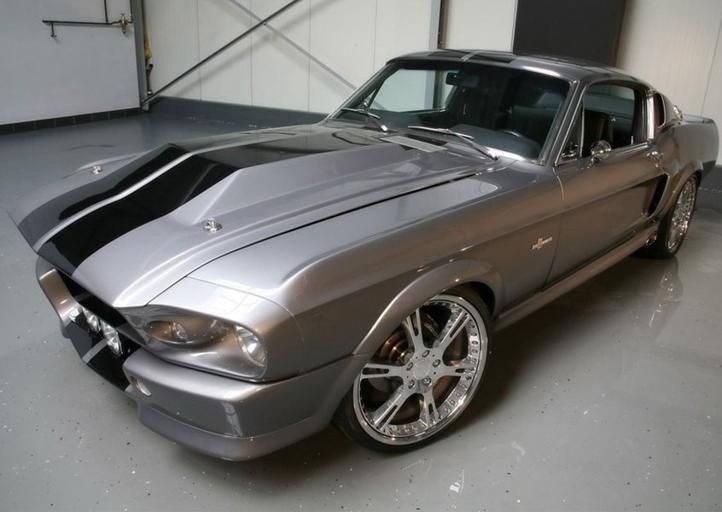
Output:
<box><xmin>3</xmin><ymin>46</ymin><xmax>718</xmax><ymax>462</ymax></box>
